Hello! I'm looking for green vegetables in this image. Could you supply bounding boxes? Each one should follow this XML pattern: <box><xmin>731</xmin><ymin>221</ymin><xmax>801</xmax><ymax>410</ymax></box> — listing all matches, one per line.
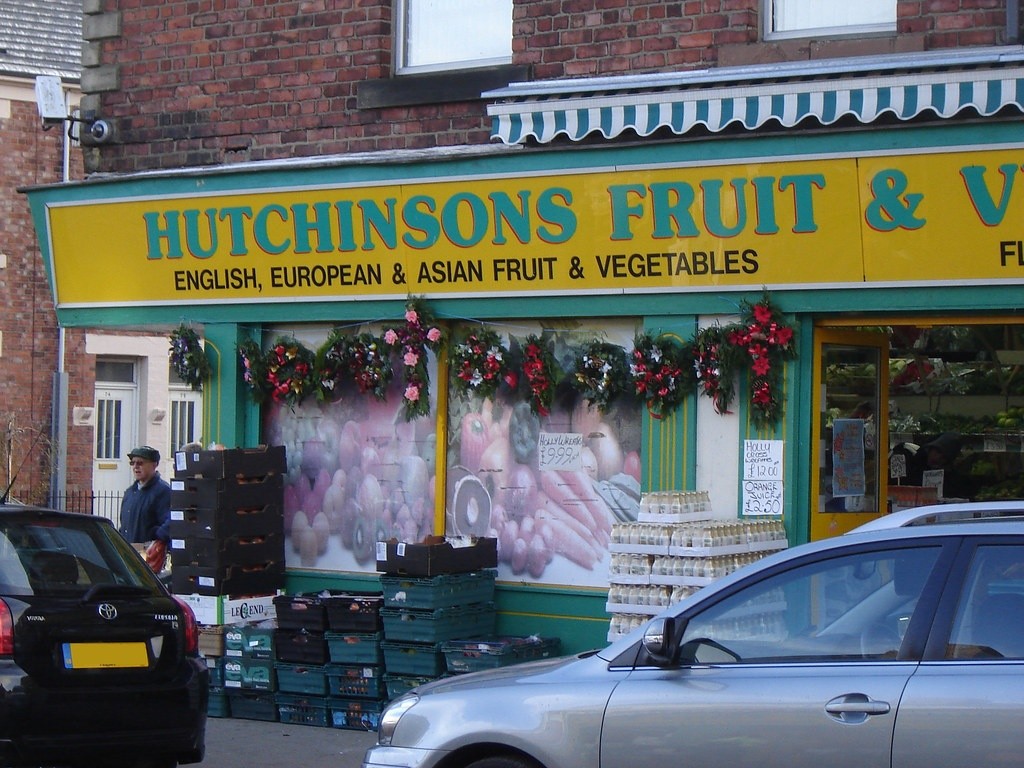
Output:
<box><xmin>888</xmin><ymin>363</ymin><xmax>1012</xmax><ymax>435</ymax></box>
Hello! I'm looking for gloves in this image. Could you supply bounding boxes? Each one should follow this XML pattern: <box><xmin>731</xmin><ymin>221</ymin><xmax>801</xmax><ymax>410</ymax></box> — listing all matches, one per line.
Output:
<box><xmin>148</xmin><ymin>540</ymin><xmax>167</xmax><ymax>575</ymax></box>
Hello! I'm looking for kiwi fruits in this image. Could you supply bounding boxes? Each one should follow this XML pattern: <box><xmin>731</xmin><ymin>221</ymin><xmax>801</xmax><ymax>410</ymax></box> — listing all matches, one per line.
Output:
<box><xmin>341</xmin><ymin>499</ymin><xmax>390</xmax><ymax>562</ymax></box>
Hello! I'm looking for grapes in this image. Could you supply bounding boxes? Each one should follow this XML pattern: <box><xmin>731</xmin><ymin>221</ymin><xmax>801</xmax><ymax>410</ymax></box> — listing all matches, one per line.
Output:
<box><xmin>273</xmin><ymin>403</ymin><xmax>311</xmax><ymax>486</ymax></box>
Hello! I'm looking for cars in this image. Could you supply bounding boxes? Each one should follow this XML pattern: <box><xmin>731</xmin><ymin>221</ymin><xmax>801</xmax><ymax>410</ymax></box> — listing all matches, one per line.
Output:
<box><xmin>0</xmin><ymin>501</ymin><xmax>209</xmax><ymax>768</ymax></box>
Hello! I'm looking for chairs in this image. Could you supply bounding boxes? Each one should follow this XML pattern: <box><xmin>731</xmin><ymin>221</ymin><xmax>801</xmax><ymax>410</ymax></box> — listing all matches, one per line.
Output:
<box><xmin>31</xmin><ymin>551</ymin><xmax>80</xmax><ymax>584</ymax></box>
<box><xmin>972</xmin><ymin>592</ymin><xmax>1023</xmax><ymax>663</ymax></box>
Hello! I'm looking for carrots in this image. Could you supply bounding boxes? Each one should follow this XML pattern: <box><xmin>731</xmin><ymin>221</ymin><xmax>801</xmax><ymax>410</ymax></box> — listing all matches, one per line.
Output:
<box><xmin>534</xmin><ymin>464</ymin><xmax>618</xmax><ymax>568</ymax></box>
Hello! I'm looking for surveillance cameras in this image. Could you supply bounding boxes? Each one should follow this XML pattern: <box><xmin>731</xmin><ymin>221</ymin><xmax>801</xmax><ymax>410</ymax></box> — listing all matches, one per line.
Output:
<box><xmin>35</xmin><ymin>76</ymin><xmax>68</xmax><ymax>127</ymax></box>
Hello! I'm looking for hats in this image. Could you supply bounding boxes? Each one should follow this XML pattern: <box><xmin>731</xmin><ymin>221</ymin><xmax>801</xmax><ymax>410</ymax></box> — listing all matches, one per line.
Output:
<box><xmin>127</xmin><ymin>446</ymin><xmax>160</xmax><ymax>461</ymax></box>
<box><xmin>920</xmin><ymin>432</ymin><xmax>961</xmax><ymax>462</ymax></box>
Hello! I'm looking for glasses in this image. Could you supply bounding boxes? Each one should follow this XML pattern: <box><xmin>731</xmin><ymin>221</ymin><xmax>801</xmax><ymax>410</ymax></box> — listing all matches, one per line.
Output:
<box><xmin>129</xmin><ymin>461</ymin><xmax>148</xmax><ymax>465</ymax></box>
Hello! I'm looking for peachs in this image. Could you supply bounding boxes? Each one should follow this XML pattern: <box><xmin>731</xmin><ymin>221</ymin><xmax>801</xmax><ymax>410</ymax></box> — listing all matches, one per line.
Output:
<box><xmin>323</xmin><ymin>420</ymin><xmax>382</xmax><ymax>529</ymax></box>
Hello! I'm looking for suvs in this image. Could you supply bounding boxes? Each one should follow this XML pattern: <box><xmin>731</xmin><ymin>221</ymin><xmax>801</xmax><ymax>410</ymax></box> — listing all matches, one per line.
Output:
<box><xmin>361</xmin><ymin>499</ymin><xmax>1023</xmax><ymax>768</ymax></box>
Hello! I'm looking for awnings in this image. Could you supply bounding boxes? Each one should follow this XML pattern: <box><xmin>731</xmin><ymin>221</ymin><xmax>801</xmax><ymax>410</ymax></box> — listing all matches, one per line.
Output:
<box><xmin>483</xmin><ymin>42</ymin><xmax>1024</xmax><ymax>146</ymax></box>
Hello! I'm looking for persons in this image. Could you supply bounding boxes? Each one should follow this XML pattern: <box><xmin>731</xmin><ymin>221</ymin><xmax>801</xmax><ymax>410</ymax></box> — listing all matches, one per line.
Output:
<box><xmin>118</xmin><ymin>445</ymin><xmax>173</xmax><ymax>576</ymax></box>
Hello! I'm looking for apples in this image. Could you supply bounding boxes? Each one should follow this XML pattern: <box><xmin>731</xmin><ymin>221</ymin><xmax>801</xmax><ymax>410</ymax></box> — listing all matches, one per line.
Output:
<box><xmin>477</xmin><ymin>397</ymin><xmax>541</xmax><ymax>505</ymax></box>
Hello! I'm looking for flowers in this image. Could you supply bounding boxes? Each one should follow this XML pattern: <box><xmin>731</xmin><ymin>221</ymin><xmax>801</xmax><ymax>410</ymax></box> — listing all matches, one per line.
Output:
<box><xmin>164</xmin><ymin>294</ymin><xmax>797</xmax><ymax>433</ymax></box>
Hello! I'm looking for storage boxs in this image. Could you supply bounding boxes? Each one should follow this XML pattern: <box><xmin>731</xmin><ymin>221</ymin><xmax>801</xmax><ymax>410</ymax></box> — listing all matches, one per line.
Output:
<box><xmin>170</xmin><ymin>446</ymin><xmax>562</xmax><ymax>735</ymax></box>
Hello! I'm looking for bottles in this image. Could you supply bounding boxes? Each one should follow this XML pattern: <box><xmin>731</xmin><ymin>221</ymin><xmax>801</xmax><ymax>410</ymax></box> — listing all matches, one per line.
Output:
<box><xmin>638</xmin><ymin>488</ymin><xmax>711</xmax><ymax>515</ymax></box>
<box><xmin>608</xmin><ymin>519</ymin><xmax>786</xmax><ymax>548</ymax></box>
<box><xmin>607</xmin><ymin>550</ymin><xmax>785</xmax><ymax>578</ymax></box>
<box><xmin>606</xmin><ymin>585</ymin><xmax>786</xmax><ymax>610</ymax></box>
<box><xmin>607</xmin><ymin>611</ymin><xmax>788</xmax><ymax>645</ymax></box>
<box><xmin>893</xmin><ymin>485</ymin><xmax>974</xmax><ymax>518</ymax></box>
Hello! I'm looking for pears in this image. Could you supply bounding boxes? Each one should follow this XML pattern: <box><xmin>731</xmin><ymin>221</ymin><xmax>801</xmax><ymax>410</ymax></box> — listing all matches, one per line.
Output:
<box><xmin>586</xmin><ymin>422</ymin><xmax>623</xmax><ymax>480</ymax></box>
<box><xmin>995</xmin><ymin>406</ymin><xmax>1024</xmax><ymax>428</ymax></box>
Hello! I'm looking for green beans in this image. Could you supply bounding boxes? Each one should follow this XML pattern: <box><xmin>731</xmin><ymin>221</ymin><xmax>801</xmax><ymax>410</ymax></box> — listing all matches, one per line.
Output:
<box><xmin>590</xmin><ymin>474</ymin><xmax>641</xmax><ymax>523</ymax></box>
